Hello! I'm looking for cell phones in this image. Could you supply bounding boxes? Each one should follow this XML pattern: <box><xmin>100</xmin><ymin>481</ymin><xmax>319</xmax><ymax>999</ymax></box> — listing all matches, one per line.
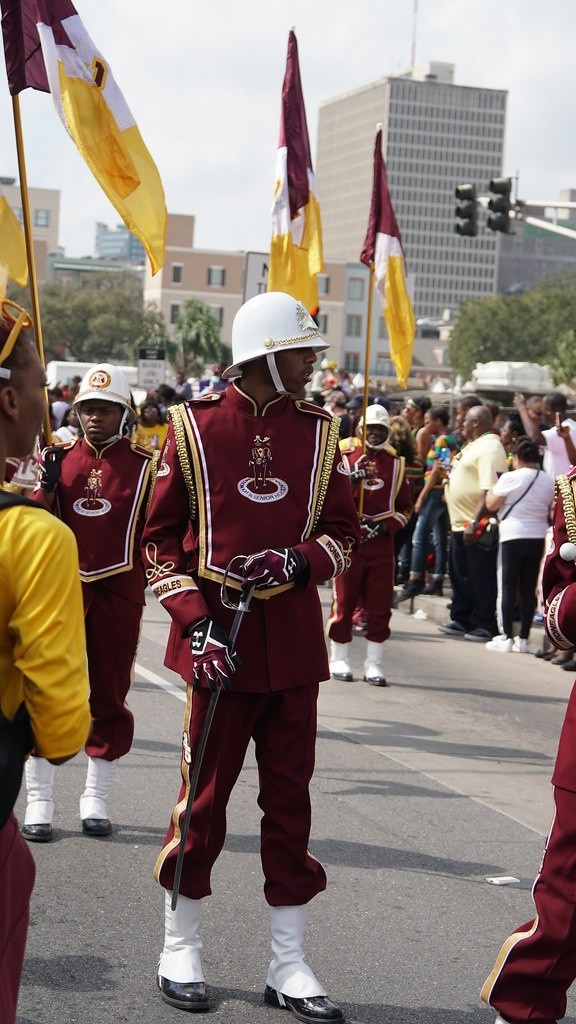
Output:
<box><xmin>557</xmin><ymin>412</ymin><xmax>563</xmax><ymax>431</ymax></box>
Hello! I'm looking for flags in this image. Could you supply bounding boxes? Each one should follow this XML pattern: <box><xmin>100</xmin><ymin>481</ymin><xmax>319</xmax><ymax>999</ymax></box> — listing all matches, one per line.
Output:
<box><xmin>0</xmin><ymin>0</ymin><xmax>166</xmax><ymax>274</ymax></box>
<box><xmin>267</xmin><ymin>29</ymin><xmax>325</xmax><ymax>318</ymax></box>
<box><xmin>360</xmin><ymin>131</ymin><xmax>415</xmax><ymax>390</ymax></box>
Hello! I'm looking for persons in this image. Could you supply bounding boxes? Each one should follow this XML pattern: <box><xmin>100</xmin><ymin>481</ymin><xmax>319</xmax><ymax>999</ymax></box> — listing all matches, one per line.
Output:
<box><xmin>480</xmin><ymin>466</ymin><xmax>576</xmax><ymax>1024</ymax></box>
<box><xmin>20</xmin><ymin>363</ymin><xmax>154</xmax><ymax>840</ymax></box>
<box><xmin>0</xmin><ymin>311</ymin><xmax>90</xmax><ymax>1023</ymax></box>
<box><xmin>338</xmin><ymin>403</ymin><xmax>412</xmax><ymax>686</ymax></box>
<box><xmin>142</xmin><ymin>291</ymin><xmax>361</xmax><ymax>1023</ymax></box>
<box><xmin>0</xmin><ymin>369</ymin><xmax>576</xmax><ymax>672</ymax></box>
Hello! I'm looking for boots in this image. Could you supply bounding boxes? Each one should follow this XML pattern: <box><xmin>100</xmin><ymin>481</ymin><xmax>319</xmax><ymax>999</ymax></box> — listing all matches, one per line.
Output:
<box><xmin>156</xmin><ymin>889</ymin><xmax>210</xmax><ymax>1009</ymax></box>
<box><xmin>330</xmin><ymin>641</ymin><xmax>353</xmax><ymax>681</ymax></box>
<box><xmin>395</xmin><ymin>579</ymin><xmax>422</xmax><ymax>602</ymax></box>
<box><xmin>265</xmin><ymin>902</ymin><xmax>344</xmax><ymax>1024</ymax></box>
<box><xmin>21</xmin><ymin>755</ymin><xmax>54</xmax><ymax>838</ymax></box>
<box><xmin>421</xmin><ymin>576</ymin><xmax>445</xmax><ymax>596</ymax></box>
<box><xmin>365</xmin><ymin>639</ymin><xmax>386</xmax><ymax>686</ymax></box>
<box><xmin>79</xmin><ymin>754</ymin><xmax>114</xmax><ymax>837</ymax></box>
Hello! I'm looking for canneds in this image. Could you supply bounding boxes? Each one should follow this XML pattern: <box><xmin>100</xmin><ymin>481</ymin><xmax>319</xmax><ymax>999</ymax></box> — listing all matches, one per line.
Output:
<box><xmin>440</xmin><ymin>448</ymin><xmax>451</xmax><ymax>469</ymax></box>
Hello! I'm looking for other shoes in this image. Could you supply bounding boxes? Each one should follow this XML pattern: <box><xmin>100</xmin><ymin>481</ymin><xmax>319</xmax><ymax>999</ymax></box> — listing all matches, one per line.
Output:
<box><xmin>513</xmin><ymin>636</ymin><xmax>529</xmax><ymax>653</ymax></box>
<box><xmin>483</xmin><ymin>638</ymin><xmax>514</xmax><ymax>652</ymax></box>
<box><xmin>461</xmin><ymin>627</ymin><xmax>495</xmax><ymax>642</ymax></box>
<box><xmin>437</xmin><ymin>621</ymin><xmax>466</xmax><ymax>635</ymax></box>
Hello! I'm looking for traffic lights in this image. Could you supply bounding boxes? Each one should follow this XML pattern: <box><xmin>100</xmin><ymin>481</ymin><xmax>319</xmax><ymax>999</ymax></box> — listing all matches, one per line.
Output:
<box><xmin>450</xmin><ymin>181</ymin><xmax>478</xmax><ymax>237</ymax></box>
<box><xmin>486</xmin><ymin>180</ymin><xmax>513</xmax><ymax>235</ymax></box>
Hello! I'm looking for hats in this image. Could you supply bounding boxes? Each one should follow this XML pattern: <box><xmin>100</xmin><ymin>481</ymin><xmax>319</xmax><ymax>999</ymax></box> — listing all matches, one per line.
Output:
<box><xmin>324</xmin><ymin>391</ymin><xmax>345</xmax><ymax>403</ymax></box>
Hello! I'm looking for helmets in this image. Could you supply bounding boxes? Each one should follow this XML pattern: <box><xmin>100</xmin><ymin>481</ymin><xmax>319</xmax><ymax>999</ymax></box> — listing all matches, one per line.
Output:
<box><xmin>222</xmin><ymin>292</ymin><xmax>332</xmax><ymax>377</ymax></box>
<box><xmin>358</xmin><ymin>405</ymin><xmax>392</xmax><ymax>437</ymax></box>
<box><xmin>72</xmin><ymin>364</ymin><xmax>137</xmax><ymax>426</ymax></box>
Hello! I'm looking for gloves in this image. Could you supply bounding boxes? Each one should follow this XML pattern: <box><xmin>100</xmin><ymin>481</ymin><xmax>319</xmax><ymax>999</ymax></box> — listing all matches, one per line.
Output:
<box><xmin>347</xmin><ymin>454</ymin><xmax>364</xmax><ymax>484</ymax></box>
<box><xmin>37</xmin><ymin>433</ymin><xmax>64</xmax><ymax>494</ymax></box>
<box><xmin>238</xmin><ymin>546</ymin><xmax>308</xmax><ymax>593</ymax></box>
<box><xmin>183</xmin><ymin>621</ymin><xmax>237</xmax><ymax>691</ymax></box>
<box><xmin>357</xmin><ymin>515</ymin><xmax>386</xmax><ymax>544</ymax></box>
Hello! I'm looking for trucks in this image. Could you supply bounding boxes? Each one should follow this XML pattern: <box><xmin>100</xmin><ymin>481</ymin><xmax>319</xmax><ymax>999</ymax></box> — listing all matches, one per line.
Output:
<box><xmin>44</xmin><ymin>360</ymin><xmax>147</xmax><ymax>407</ymax></box>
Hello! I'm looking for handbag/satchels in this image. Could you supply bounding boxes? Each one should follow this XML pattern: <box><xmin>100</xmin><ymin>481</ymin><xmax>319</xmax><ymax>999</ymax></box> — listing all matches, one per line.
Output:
<box><xmin>478</xmin><ymin>521</ymin><xmax>501</xmax><ymax>548</ymax></box>
